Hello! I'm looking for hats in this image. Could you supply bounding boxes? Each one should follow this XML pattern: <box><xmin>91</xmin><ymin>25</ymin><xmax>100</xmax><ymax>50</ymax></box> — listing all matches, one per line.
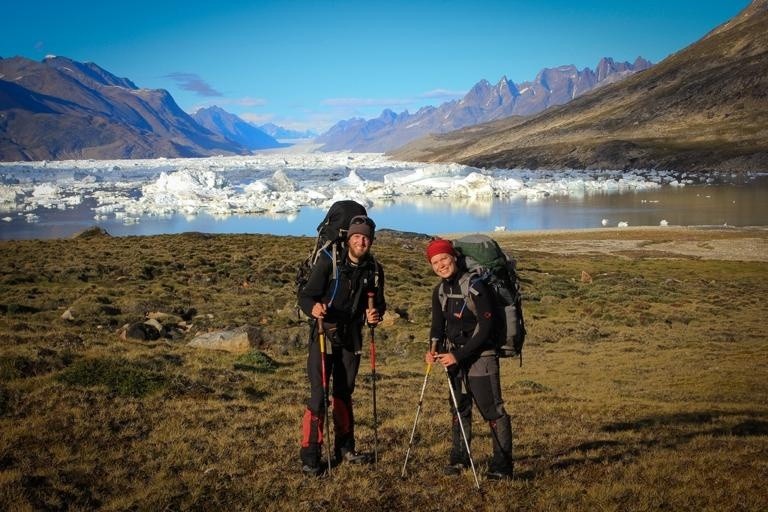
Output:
<box><xmin>346</xmin><ymin>215</ymin><xmax>374</xmax><ymax>244</ymax></box>
<box><xmin>426</xmin><ymin>239</ymin><xmax>453</xmax><ymax>263</ymax></box>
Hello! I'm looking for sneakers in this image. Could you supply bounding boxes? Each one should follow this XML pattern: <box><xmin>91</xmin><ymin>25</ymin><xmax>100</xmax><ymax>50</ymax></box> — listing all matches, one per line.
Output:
<box><xmin>443</xmin><ymin>461</ymin><xmax>464</xmax><ymax>476</ymax></box>
<box><xmin>302</xmin><ymin>463</ymin><xmax>320</xmax><ymax>476</ymax></box>
<box><xmin>482</xmin><ymin>471</ymin><xmax>512</xmax><ymax>483</ymax></box>
<box><xmin>334</xmin><ymin>447</ymin><xmax>366</xmax><ymax>465</ymax></box>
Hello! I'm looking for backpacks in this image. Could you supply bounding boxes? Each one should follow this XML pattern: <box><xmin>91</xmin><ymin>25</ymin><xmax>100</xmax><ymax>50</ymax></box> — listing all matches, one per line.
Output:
<box><xmin>291</xmin><ymin>199</ymin><xmax>379</xmax><ymax>356</ymax></box>
<box><xmin>438</xmin><ymin>234</ymin><xmax>526</xmax><ymax>367</ymax></box>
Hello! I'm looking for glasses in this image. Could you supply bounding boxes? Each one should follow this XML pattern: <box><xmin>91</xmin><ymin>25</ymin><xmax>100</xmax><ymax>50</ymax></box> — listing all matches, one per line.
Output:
<box><xmin>350</xmin><ymin>217</ymin><xmax>375</xmax><ymax>228</ymax></box>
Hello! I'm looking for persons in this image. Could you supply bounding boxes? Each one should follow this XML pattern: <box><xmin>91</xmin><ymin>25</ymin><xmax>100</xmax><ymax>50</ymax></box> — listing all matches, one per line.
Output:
<box><xmin>425</xmin><ymin>239</ymin><xmax>514</xmax><ymax>481</ymax></box>
<box><xmin>296</xmin><ymin>216</ymin><xmax>387</xmax><ymax>477</ymax></box>
